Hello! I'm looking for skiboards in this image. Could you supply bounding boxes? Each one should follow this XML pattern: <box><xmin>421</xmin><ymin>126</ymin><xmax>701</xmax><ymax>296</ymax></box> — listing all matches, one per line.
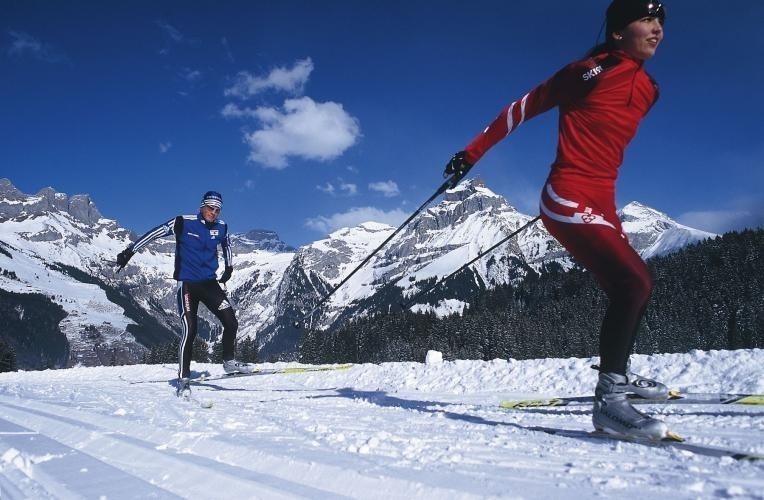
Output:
<box><xmin>500</xmin><ymin>394</ymin><xmax>764</xmax><ymax>464</ymax></box>
<box><xmin>119</xmin><ymin>361</ymin><xmax>352</xmax><ymax>408</ymax></box>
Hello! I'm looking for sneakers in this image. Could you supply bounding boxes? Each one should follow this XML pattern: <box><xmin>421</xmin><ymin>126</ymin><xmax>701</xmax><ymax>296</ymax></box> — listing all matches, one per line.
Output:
<box><xmin>176</xmin><ymin>377</ymin><xmax>191</xmax><ymax>398</ymax></box>
<box><xmin>223</xmin><ymin>358</ymin><xmax>254</xmax><ymax>374</ymax></box>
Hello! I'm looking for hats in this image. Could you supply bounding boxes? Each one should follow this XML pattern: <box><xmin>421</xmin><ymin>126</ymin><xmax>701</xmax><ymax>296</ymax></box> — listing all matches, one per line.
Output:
<box><xmin>200</xmin><ymin>190</ymin><xmax>223</xmax><ymax>210</ymax></box>
<box><xmin>605</xmin><ymin>0</ymin><xmax>665</xmax><ymax>34</ymax></box>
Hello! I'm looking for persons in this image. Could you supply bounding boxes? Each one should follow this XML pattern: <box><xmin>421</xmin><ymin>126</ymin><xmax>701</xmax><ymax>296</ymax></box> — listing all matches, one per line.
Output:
<box><xmin>443</xmin><ymin>0</ymin><xmax>670</xmax><ymax>443</ymax></box>
<box><xmin>114</xmin><ymin>190</ymin><xmax>257</xmax><ymax>398</ymax></box>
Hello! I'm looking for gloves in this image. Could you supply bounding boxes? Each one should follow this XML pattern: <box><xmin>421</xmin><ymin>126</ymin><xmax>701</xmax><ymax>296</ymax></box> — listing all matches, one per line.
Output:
<box><xmin>444</xmin><ymin>151</ymin><xmax>475</xmax><ymax>189</ymax></box>
<box><xmin>219</xmin><ymin>266</ymin><xmax>233</xmax><ymax>283</ymax></box>
<box><xmin>116</xmin><ymin>248</ymin><xmax>135</xmax><ymax>268</ymax></box>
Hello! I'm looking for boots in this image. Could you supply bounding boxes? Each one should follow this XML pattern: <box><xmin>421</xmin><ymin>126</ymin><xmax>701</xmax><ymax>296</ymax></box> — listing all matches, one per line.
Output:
<box><xmin>591</xmin><ymin>370</ymin><xmax>667</xmax><ymax>441</ymax></box>
<box><xmin>625</xmin><ymin>356</ymin><xmax>666</xmax><ymax>400</ymax></box>
